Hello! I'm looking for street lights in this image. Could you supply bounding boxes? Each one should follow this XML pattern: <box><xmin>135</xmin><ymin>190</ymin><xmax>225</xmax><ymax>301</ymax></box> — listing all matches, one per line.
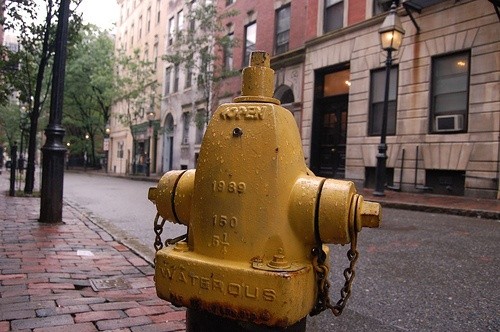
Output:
<box><xmin>66</xmin><ymin>139</ymin><xmax>71</xmax><ymax>170</ymax></box>
<box><xmin>84</xmin><ymin>132</ymin><xmax>90</xmax><ymax>171</ymax></box>
<box><xmin>146</xmin><ymin>103</ymin><xmax>156</xmax><ymax>176</ymax></box>
<box><xmin>372</xmin><ymin>2</ymin><xmax>405</xmax><ymax>196</ymax></box>
<box><xmin>105</xmin><ymin>121</ymin><xmax>111</xmax><ymax>173</ymax></box>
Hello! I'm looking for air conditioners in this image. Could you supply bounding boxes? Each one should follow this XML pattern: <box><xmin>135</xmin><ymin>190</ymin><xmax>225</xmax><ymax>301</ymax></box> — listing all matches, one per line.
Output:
<box><xmin>435</xmin><ymin>114</ymin><xmax>464</xmax><ymax>132</ymax></box>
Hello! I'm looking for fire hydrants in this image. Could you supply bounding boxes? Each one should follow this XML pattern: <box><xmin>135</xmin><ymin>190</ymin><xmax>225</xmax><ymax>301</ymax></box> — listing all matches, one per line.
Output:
<box><xmin>148</xmin><ymin>50</ymin><xmax>380</xmax><ymax>332</ymax></box>
